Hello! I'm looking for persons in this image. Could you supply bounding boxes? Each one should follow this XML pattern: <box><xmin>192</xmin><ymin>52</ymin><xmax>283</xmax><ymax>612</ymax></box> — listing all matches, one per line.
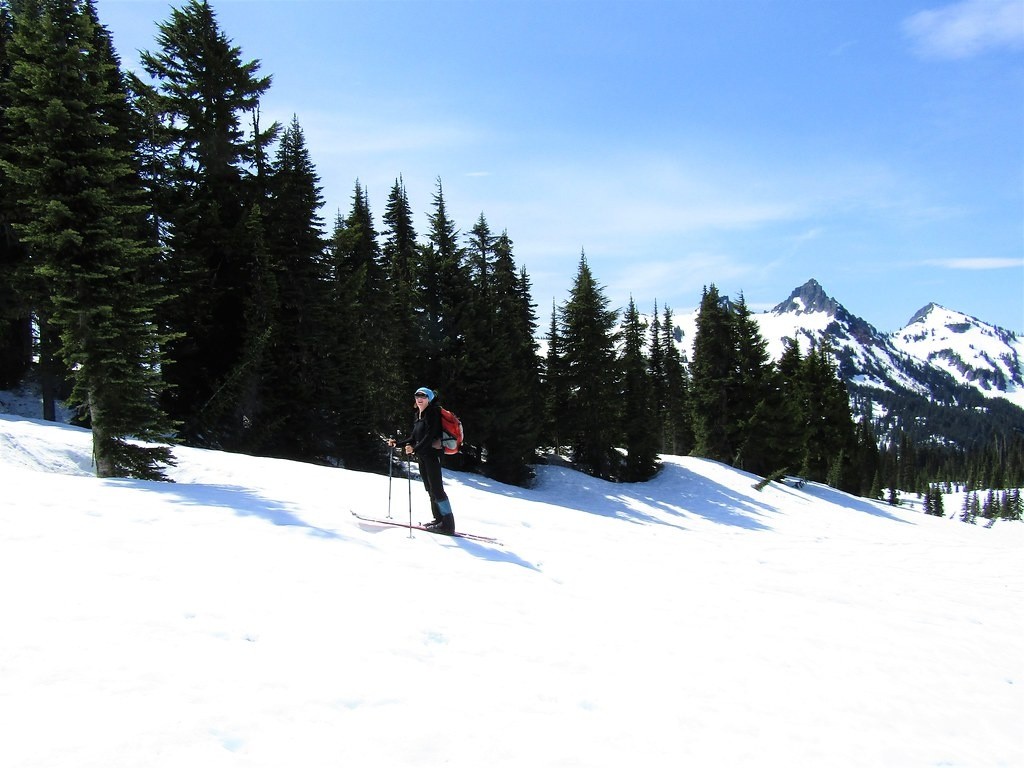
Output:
<box><xmin>387</xmin><ymin>387</ymin><xmax>455</xmax><ymax>536</ymax></box>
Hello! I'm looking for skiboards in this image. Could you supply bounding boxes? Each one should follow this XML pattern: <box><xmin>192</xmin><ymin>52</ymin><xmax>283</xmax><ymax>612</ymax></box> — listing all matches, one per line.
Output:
<box><xmin>349</xmin><ymin>505</ymin><xmax>505</xmax><ymax>547</ymax></box>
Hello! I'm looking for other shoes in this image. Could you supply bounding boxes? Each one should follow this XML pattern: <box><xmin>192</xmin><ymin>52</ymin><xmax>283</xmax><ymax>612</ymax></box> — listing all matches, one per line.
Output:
<box><xmin>426</xmin><ymin>525</ymin><xmax>455</xmax><ymax>536</ymax></box>
<box><xmin>423</xmin><ymin>520</ymin><xmax>441</xmax><ymax>527</ymax></box>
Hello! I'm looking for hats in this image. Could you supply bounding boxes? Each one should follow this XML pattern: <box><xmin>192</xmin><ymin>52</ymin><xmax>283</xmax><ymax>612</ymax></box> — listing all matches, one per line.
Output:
<box><xmin>415</xmin><ymin>387</ymin><xmax>435</xmax><ymax>403</ymax></box>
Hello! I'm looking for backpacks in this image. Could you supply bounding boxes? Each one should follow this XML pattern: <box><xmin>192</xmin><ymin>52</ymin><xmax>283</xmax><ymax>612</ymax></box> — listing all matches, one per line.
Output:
<box><xmin>423</xmin><ymin>406</ymin><xmax>464</xmax><ymax>455</ymax></box>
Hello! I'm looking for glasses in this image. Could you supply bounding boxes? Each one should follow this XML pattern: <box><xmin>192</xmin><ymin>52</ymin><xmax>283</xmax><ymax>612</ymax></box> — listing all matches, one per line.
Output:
<box><xmin>415</xmin><ymin>393</ymin><xmax>428</xmax><ymax>399</ymax></box>
